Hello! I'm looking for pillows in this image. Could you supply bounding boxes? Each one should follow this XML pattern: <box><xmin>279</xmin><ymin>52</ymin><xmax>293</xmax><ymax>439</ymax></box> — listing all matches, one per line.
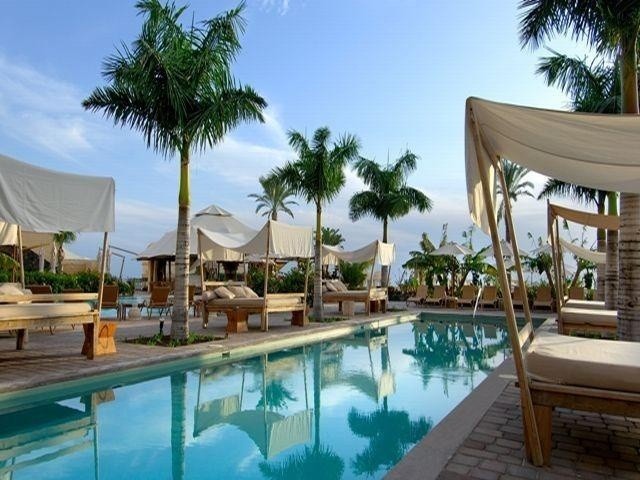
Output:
<box><xmin>322</xmin><ymin>279</ymin><xmax>349</xmax><ymax>294</ymax></box>
<box><xmin>204</xmin><ymin>284</ymin><xmax>257</xmax><ymax>303</ymax></box>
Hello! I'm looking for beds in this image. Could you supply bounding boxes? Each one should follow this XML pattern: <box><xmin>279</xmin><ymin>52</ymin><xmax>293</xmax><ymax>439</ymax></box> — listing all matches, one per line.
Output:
<box><xmin>1</xmin><ymin>393</ymin><xmax>103</xmax><ymax>479</ymax></box>
<box><xmin>0</xmin><ymin>153</ymin><xmax>117</xmax><ymax>363</ymax></box>
<box><xmin>316</xmin><ymin>238</ymin><xmax>396</xmax><ymax>316</ymax></box>
<box><xmin>466</xmin><ymin>95</ymin><xmax>640</xmax><ymax>469</ymax></box>
<box><xmin>196</xmin><ymin>220</ymin><xmax>313</xmax><ymax>330</ymax></box>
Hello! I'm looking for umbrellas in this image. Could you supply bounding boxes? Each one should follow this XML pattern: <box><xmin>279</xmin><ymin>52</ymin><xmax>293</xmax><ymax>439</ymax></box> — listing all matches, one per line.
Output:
<box><xmin>426</xmin><ymin>241</ymin><xmax>477</xmax><ymax>298</ymax></box>
<box><xmin>477</xmin><ymin>238</ymin><xmax>530</xmax><ymax>259</ymax></box>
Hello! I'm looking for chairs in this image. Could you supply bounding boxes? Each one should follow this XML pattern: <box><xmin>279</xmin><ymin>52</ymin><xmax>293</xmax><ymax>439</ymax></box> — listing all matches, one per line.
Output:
<box><xmin>409</xmin><ymin>284</ymin><xmax>587</xmax><ymax>314</ymax></box>
<box><xmin>35</xmin><ymin>279</ymin><xmax>199</xmax><ymax>333</ymax></box>
